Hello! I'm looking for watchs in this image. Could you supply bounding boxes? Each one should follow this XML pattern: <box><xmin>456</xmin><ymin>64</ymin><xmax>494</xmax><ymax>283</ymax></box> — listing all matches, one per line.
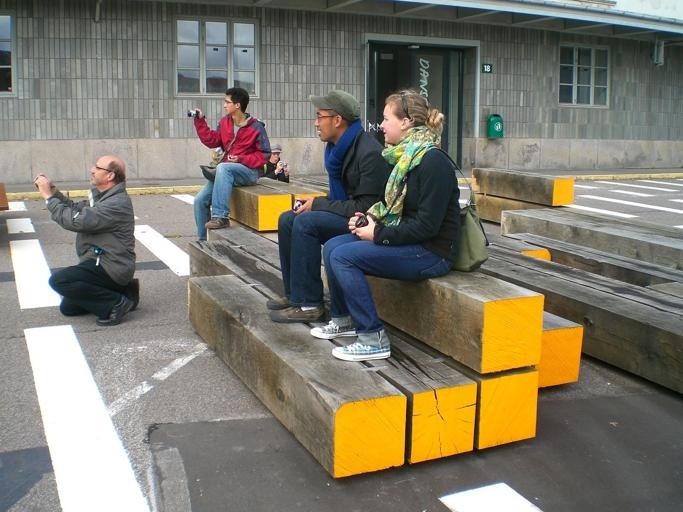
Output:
<box><xmin>45</xmin><ymin>196</ymin><xmax>53</xmax><ymax>204</ymax></box>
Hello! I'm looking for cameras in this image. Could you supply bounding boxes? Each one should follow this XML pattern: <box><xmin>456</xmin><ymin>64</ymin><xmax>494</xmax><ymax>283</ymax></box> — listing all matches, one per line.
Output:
<box><xmin>294</xmin><ymin>200</ymin><xmax>307</xmax><ymax>212</ymax></box>
<box><xmin>187</xmin><ymin>110</ymin><xmax>199</xmax><ymax>119</ymax></box>
<box><xmin>355</xmin><ymin>216</ymin><xmax>368</xmax><ymax>228</ymax></box>
<box><xmin>282</xmin><ymin>162</ymin><xmax>288</xmax><ymax>168</ymax></box>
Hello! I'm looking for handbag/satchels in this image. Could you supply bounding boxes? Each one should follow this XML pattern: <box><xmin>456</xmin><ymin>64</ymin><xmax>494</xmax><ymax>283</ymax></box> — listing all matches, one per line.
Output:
<box><xmin>454</xmin><ymin>162</ymin><xmax>489</xmax><ymax>272</ymax></box>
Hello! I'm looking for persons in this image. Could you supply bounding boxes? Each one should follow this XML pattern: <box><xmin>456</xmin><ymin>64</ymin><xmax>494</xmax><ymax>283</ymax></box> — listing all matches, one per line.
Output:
<box><xmin>266</xmin><ymin>90</ymin><xmax>394</xmax><ymax>323</ymax></box>
<box><xmin>33</xmin><ymin>154</ymin><xmax>140</xmax><ymax>327</ymax></box>
<box><xmin>310</xmin><ymin>89</ymin><xmax>462</xmax><ymax>362</ymax></box>
<box><xmin>192</xmin><ymin>87</ymin><xmax>271</xmax><ymax>241</ymax></box>
<box><xmin>259</xmin><ymin>144</ymin><xmax>290</xmax><ymax>184</ymax></box>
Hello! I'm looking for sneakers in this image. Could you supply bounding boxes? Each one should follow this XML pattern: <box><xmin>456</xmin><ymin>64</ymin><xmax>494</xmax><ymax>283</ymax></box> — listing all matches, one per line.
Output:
<box><xmin>310</xmin><ymin>322</ymin><xmax>357</xmax><ymax>339</ymax></box>
<box><xmin>270</xmin><ymin>307</ymin><xmax>326</xmax><ymax>323</ymax></box>
<box><xmin>267</xmin><ymin>297</ymin><xmax>295</xmax><ymax>309</ymax></box>
<box><xmin>126</xmin><ymin>279</ymin><xmax>139</xmax><ymax>311</ymax></box>
<box><xmin>97</xmin><ymin>296</ymin><xmax>134</xmax><ymax>326</ymax></box>
<box><xmin>331</xmin><ymin>336</ymin><xmax>392</xmax><ymax>362</ymax></box>
<box><xmin>205</xmin><ymin>217</ymin><xmax>230</xmax><ymax>229</ymax></box>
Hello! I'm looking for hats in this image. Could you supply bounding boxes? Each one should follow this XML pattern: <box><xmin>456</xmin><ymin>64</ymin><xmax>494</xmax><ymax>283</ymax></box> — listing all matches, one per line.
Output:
<box><xmin>270</xmin><ymin>143</ymin><xmax>283</xmax><ymax>153</ymax></box>
<box><xmin>310</xmin><ymin>90</ymin><xmax>361</xmax><ymax>122</ymax></box>
